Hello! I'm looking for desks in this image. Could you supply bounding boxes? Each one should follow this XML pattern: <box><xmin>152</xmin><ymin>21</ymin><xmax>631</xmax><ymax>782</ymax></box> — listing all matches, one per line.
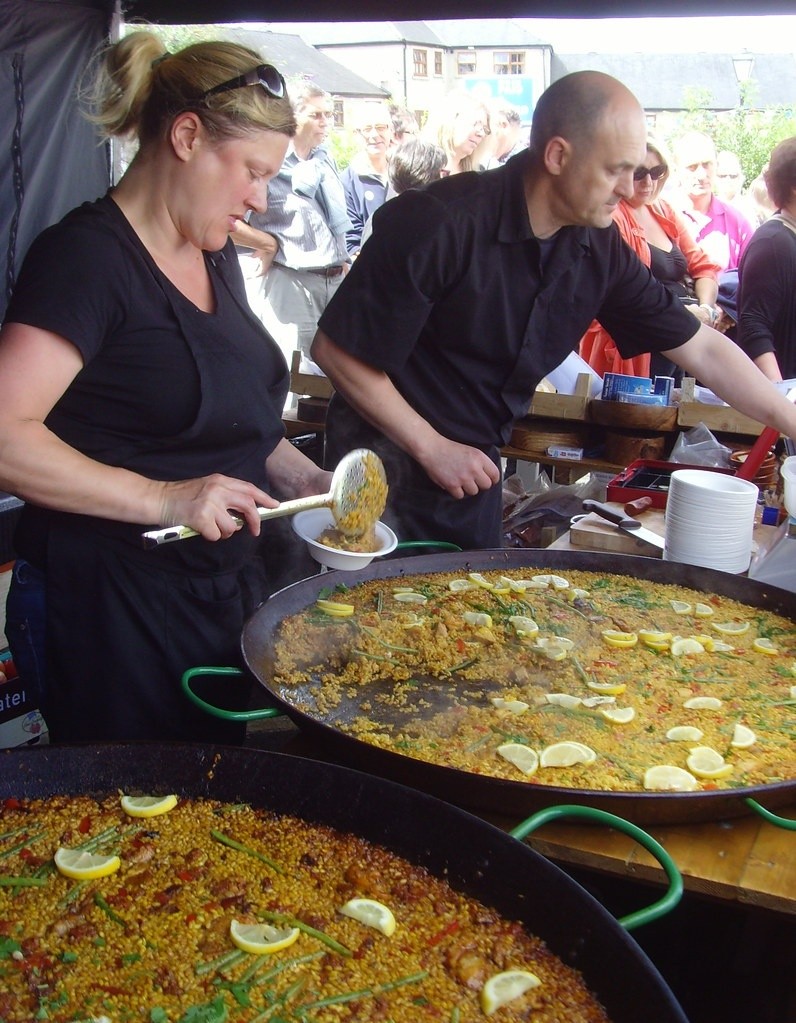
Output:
<box><xmin>362</xmin><ymin>496</ymin><xmax>796</xmax><ymax>916</ymax></box>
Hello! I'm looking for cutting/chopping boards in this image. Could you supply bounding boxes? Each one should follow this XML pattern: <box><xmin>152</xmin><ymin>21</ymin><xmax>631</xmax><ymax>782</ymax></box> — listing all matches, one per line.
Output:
<box><xmin>568</xmin><ymin>496</ymin><xmax>787</xmax><ymax>577</ymax></box>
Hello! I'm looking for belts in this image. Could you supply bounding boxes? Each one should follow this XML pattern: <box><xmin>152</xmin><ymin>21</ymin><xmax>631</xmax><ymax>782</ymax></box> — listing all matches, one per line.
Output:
<box><xmin>306</xmin><ymin>265</ymin><xmax>344</xmax><ymax>277</ymax></box>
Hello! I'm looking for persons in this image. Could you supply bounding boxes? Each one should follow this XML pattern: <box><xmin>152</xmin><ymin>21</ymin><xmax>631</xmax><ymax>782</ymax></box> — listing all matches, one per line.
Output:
<box><xmin>229</xmin><ymin>78</ymin><xmax>529</xmax><ymax>409</ymax></box>
<box><xmin>573</xmin><ymin>135</ymin><xmax>796</xmax><ymax>388</ymax></box>
<box><xmin>310</xmin><ymin>67</ymin><xmax>796</xmax><ymax>570</ymax></box>
<box><xmin>0</xmin><ymin>31</ymin><xmax>336</xmax><ymax>744</ymax></box>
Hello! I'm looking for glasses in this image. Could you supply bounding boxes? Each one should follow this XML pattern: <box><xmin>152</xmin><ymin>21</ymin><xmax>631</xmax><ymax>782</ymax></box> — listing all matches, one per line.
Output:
<box><xmin>634</xmin><ymin>164</ymin><xmax>668</xmax><ymax>181</ymax></box>
<box><xmin>717</xmin><ymin>173</ymin><xmax>738</xmax><ymax>179</ymax></box>
<box><xmin>300</xmin><ymin>112</ymin><xmax>334</xmax><ymax>119</ymax></box>
<box><xmin>186</xmin><ymin>64</ymin><xmax>289</xmax><ymax>106</ymax></box>
<box><xmin>474</xmin><ymin>122</ymin><xmax>490</xmax><ymax>135</ymax></box>
<box><xmin>362</xmin><ymin>124</ymin><xmax>388</xmax><ymax>132</ymax></box>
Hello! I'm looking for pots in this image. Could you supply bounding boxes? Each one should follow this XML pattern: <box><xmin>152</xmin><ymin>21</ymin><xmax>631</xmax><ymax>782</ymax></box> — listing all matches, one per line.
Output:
<box><xmin>179</xmin><ymin>548</ymin><xmax>796</xmax><ymax>835</ymax></box>
<box><xmin>1</xmin><ymin>731</ymin><xmax>697</xmax><ymax>1023</ymax></box>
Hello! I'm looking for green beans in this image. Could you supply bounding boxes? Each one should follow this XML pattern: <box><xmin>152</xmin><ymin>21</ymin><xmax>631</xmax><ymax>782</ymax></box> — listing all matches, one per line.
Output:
<box><xmin>0</xmin><ymin>803</ymin><xmax>461</xmax><ymax>1023</ymax></box>
<box><xmin>304</xmin><ymin>587</ymin><xmax>796</xmax><ymax>779</ymax></box>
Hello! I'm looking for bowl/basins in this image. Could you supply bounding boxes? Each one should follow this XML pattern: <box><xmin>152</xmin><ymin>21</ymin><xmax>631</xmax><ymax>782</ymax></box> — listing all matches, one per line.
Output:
<box><xmin>292</xmin><ymin>508</ymin><xmax>398</xmax><ymax>571</ymax></box>
<box><xmin>731</xmin><ymin>448</ymin><xmax>780</xmax><ymax>495</ymax></box>
<box><xmin>660</xmin><ymin>468</ymin><xmax>760</xmax><ymax>576</ymax></box>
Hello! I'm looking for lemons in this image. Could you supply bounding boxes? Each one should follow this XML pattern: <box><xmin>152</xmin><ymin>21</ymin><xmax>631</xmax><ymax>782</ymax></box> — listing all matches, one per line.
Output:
<box><xmin>121</xmin><ymin>794</ymin><xmax>178</xmax><ymax>818</ymax></box>
<box><xmin>338</xmin><ymin>897</ymin><xmax>396</xmax><ymax>937</ymax></box>
<box><xmin>54</xmin><ymin>847</ymin><xmax>121</xmax><ymax>879</ymax></box>
<box><xmin>230</xmin><ymin>918</ymin><xmax>301</xmax><ymax>954</ymax></box>
<box><xmin>480</xmin><ymin>970</ymin><xmax>542</xmax><ymax>1015</ymax></box>
<box><xmin>317</xmin><ymin>575</ymin><xmax>779</xmax><ymax>790</ymax></box>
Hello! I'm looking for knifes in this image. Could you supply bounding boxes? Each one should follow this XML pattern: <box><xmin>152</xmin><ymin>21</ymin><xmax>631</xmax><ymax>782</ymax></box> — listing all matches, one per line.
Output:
<box><xmin>582</xmin><ymin>498</ymin><xmax>665</xmax><ymax>551</ymax></box>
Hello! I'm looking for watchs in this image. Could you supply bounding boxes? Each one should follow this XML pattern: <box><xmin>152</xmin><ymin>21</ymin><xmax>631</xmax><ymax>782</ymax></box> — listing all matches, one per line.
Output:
<box><xmin>700</xmin><ymin>305</ymin><xmax>719</xmax><ymax>324</ymax></box>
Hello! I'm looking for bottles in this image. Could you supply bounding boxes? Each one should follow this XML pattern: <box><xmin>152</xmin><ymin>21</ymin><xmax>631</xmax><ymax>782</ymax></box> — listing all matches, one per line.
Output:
<box><xmin>753</xmin><ymin>504</ymin><xmax>780</xmax><ymax>529</ymax></box>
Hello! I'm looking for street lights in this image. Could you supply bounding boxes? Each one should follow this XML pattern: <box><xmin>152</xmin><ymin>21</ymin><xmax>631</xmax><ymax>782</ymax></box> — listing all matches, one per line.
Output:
<box><xmin>730</xmin><ymin>46</ymin><xmax>755</xmax><ymax>134</ymax></box>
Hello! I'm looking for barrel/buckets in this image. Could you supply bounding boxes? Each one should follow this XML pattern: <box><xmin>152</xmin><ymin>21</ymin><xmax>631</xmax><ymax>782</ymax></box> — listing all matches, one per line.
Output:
<box><xmin>779</xmin><ymin>455</ymin><xmax>796</xmax><ymax>518</ymax></box>
